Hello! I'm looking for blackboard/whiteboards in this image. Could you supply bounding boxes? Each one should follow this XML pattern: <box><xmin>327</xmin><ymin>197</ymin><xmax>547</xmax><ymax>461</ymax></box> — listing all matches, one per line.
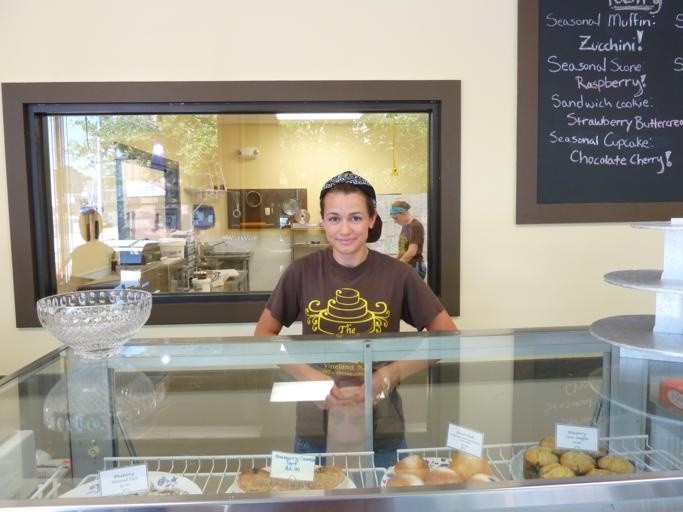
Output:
<box><xmin>516</xmin><ymin>0</ymin><xmax>683</xmax><ymax>224</ymax></box>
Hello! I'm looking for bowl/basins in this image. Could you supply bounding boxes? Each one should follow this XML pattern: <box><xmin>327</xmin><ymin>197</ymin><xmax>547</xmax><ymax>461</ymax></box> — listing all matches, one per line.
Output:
<box><xmin>36</xmin><ymin>289</ymin><xmax>153</xmax><ymax>359</ymax></box>
<box><xmin>42</xmin><ymin>354</ymin><xmax>157</xmax><ymax>434</ymax></box>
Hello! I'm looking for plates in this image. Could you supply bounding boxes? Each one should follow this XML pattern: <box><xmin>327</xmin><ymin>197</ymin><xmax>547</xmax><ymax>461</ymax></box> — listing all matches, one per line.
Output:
<box><xmin>224</xmin><ymin>464</ymin><xmax>356</xmax><ymax>489</ymax></box>
<box><xmin>282</xmin><ymin>199</ymin><xmax>298</xmax><ymax>215</ymax></box>
<box><xmin>513</xmin><ymin>445</ymin><xmax>635</xmax><ymax>481</ymax></box>
<box><xmin>380</xmin><ymin>457</ymin><xmax>495</xmax><ymax>486</ymax></box>
<box><xmin>60</xmin><ymin>472</ymin><xmax>202</xmax><ymax>495</ymax></box>
<box><xmin>294</xmin><ymin>209</ymin><xmax>310</xmax><ymax>225</ymax></box>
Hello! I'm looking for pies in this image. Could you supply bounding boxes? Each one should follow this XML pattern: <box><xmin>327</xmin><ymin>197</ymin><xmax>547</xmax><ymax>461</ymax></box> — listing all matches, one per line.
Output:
<box><xmin>238</xmin><ymin>467</ymin><xmax>344</xmax><ymax>494</ymax></box>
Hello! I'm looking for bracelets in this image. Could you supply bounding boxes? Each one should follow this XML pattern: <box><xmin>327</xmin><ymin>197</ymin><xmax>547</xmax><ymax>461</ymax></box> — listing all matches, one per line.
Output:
<box><xmin>396</xmin><ymin>363</ymin><xmax>400</xmax><ymax>386</ymax></box>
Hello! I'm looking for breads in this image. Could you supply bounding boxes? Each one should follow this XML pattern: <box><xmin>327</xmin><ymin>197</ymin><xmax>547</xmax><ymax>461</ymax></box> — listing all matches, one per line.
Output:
<box><xmin>525</xmin><ymin>436</ymin><xmax>635</xmax><ymax>479</ymax></box>
<box><xmin>386</xmin><ymin>451</ymin><xmax>496</xmax><ymax>486</ymax></box>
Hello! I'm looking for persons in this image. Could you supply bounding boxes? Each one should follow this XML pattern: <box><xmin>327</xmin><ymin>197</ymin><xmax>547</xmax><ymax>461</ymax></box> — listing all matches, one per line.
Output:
<box><xmin>389</xmin><ymin>201</ymin><xmax>426</xmax><ymax>281</ymax></box>
<box><xmin>253</xmin><ymin>171</ymin><xmax>458</xmax><ymax>488</ymax></box>
<box><xmin>68</xmin><ymin>209</ymin><xmax>121</xmax><ymax>291</ymax></box>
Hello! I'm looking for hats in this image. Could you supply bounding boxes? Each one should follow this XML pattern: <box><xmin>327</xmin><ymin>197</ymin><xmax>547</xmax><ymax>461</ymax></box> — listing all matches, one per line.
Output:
<box><xmin>389</xmin><ymin>201</ymin><xmax>410</xmax><ymax>214</ymax></box>
<box><xmin>320</xmin><ymin>171</ymin><xmax>382</xmax><ymax>243</ymax></box>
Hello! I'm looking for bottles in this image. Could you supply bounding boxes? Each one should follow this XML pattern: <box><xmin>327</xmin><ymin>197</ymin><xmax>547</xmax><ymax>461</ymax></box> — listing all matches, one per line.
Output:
<box><xmin>192</xmin><ymin>272</ymin><xmax>211</xmax><ymax>292</ymax></box>
